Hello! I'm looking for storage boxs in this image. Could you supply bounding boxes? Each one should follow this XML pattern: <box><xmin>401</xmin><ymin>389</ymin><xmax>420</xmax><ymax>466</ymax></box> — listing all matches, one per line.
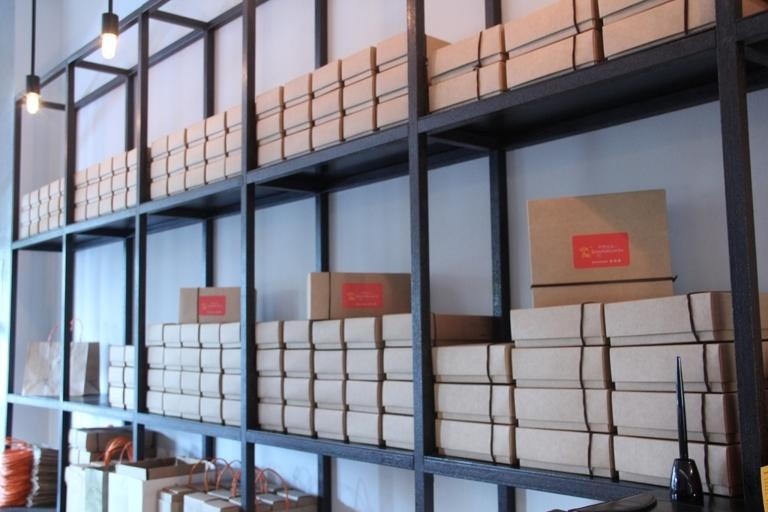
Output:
<box><xmin>176</xmin><ymin>285</ymin><xmax>256</xmax><ymax>323</ymax></box>
<box><xmin>526</xmin><ymin>189</ymin><xmax>676</xmax><ymax>305</ymax></box>
<box><xmin>310</xmin><ymin>271</ymin><xmax>412</xmax><ymax>321</ymax></box>
<box><xmin>105</xmin><ymin>455</ymin><xmax>223</xmax><ymax>512</ymax></box>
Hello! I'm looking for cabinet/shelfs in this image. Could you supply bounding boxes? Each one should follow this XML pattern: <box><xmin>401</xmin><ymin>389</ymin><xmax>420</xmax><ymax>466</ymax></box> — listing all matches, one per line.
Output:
<box><xmin>0</xmin><ymin>0</ymin><xmax>768</xmax><ymax>512</ymax></box>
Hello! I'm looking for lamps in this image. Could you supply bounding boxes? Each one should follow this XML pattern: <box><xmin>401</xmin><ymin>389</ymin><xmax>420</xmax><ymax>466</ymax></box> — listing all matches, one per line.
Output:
<box><xmin>24</xmin><ymin>0</ymin><xmax>43</xmax><ymax>117</ymax></box>
<box><xmin>96</xmin><ymin>0</ymin><xmax>122</xmax><ymax>58</ymax></box>
